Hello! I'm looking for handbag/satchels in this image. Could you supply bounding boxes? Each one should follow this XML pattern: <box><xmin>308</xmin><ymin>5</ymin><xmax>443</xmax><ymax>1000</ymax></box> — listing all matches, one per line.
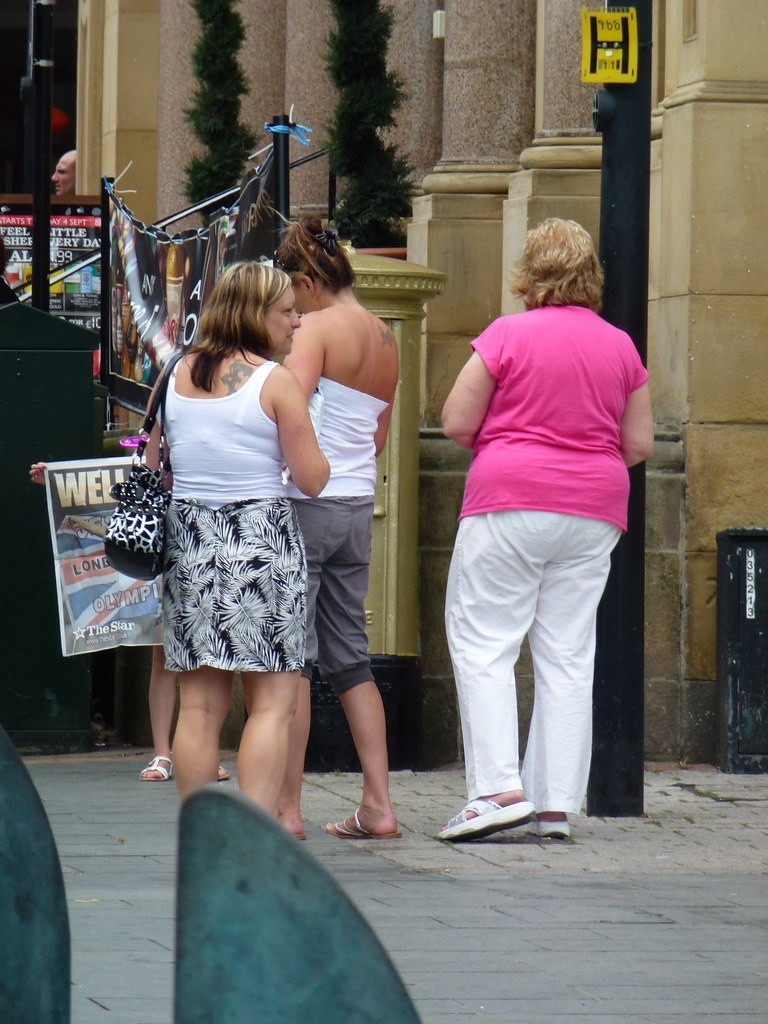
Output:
<box><xmin>101</xmin><ymin>351</ymin><xmax>186</xmax><ymax>579</ymax></box>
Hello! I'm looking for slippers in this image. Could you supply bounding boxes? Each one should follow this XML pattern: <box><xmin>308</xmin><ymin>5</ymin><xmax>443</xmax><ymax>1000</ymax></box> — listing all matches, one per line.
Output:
<box><xmin>323</xmin><ymin>809</ymin><xmax>403</xmax><ymax>840</ymax></box>
<box><xmin>288</xmin><ymin>834</ymin><xmax>306</xmax><ymax>840</ymax></box>
<box><xmin>521</xmin><ymin>810</ymin><xmax>571</xmax><ymax>837</ymax></box>
<box><xmin>435</xmin><ymin>797</ymin><xmax>536</xmax><ymax>842</ymax></box>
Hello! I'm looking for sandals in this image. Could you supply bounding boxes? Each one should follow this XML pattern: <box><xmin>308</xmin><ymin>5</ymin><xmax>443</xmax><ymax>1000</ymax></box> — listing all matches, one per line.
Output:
<box><xmin>217</xmin><ymin>766</ymin><xmax>230</xmax><ymax>779</ymax></box>
<box><xmin>139</xmin><ymin>756</ymin><xmax>174</xmax><ymax>782</ymax></box>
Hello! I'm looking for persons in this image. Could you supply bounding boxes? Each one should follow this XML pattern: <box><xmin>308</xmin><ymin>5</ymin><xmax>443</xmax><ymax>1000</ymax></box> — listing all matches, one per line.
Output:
<box><xmin>162</xmin><ymin>260</ymin><xmax>330</xmax><ymax>826</ymax></box>
<box><xmin>278</xmin><ymin>210</ymin><xmax>401</xmax><ymax>844</ymax></box>
<box><xmin>440</xmin><ymin>218</ymin><xmax>656</xmax><ymax>841</ymax></box>
<box><xmin>49</xmin><ymin>148</ymin><xmax>104</xmax><ymax>236</ymax></box>
<box><xmin>28</xmin><ymin>454</ymin><xmax>234</xmax><ymax>781</ymax></box>
